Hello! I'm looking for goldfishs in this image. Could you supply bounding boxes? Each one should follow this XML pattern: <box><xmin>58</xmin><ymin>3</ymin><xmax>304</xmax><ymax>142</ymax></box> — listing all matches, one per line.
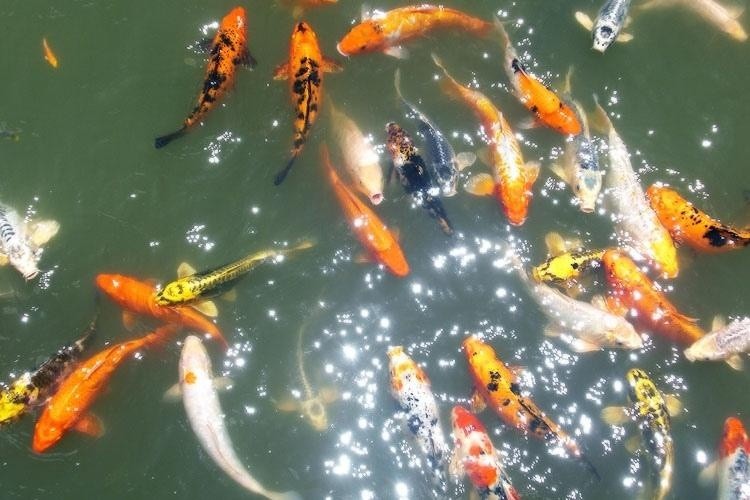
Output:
<box><xmin>267</xmin><ymin>1</ymin><xmax>750</xmax><ymax>500</ymax></box>
